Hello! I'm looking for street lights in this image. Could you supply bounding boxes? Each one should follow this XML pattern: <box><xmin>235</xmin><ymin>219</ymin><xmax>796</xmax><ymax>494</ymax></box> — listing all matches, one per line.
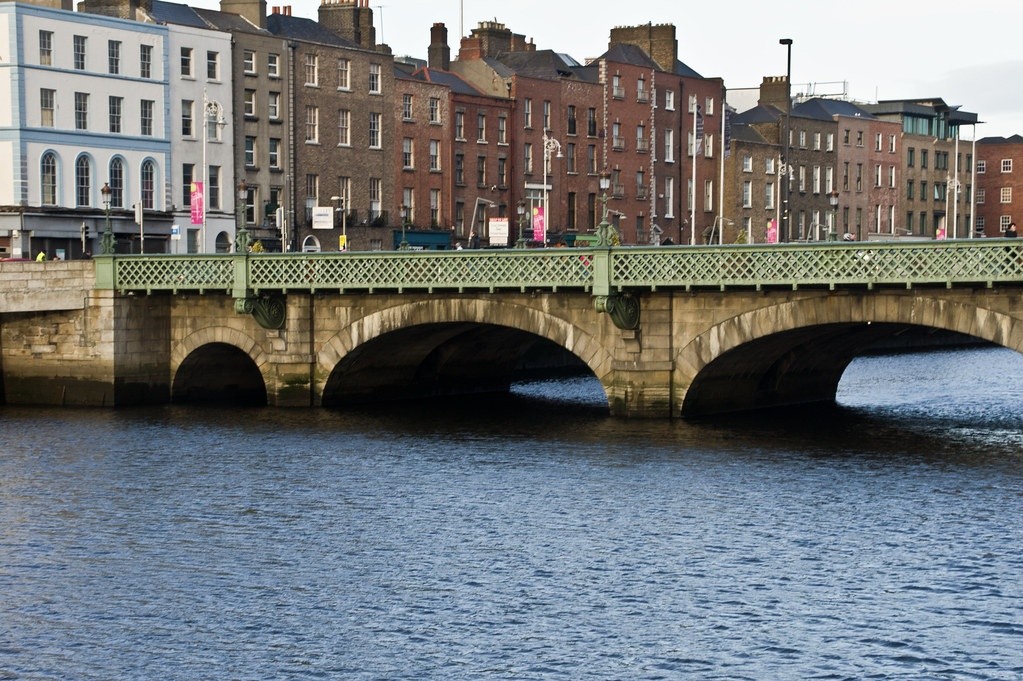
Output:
<box><xmin>202</xmin><ymin>103</ymin><xmax>229</xmax><ymax>256</ymax></box>
<box><xmin>99</xmin><ymin>182</ymin><xmax>119</xmax><ymax>255</ymax></box>
<box><xmin>776</xmin><ymin>155</ymin><xmax>796</xmax><ymax>244</ymax></box>
<box><xmin>778</xmin><ymin>35</ymin><xmax>793</xmax><ymax>245</ymax></box>
<box><xmin>514</xmin><ymin>196</ymin><xmax>528</xmax><ymax>249</ymax></box>
<box><xmin>595</xmin><ymin>168</ymin><xmax>622</xmax><ymax>246</ymax></box>
<box><xmin>331</xmin><ymin>195</ymin><xmax>348</xmax><ymax>252</ymax></box>
<box><xmin>942</xmin><ymin>169</ymin><xmax>962</xmax><ymax>242</ymax></box>
<box><xmin>543</xmin><ymin>136</ymin><xmax>565</xmax><ymax>247</ymax></box>
<box><xmin>397</xmin><ymin>203</ymin><xmax>411</xmax><ymax>251</ymax></box>
<box><xmin>236</xmin><ymin>178</ymin><xmax>252</xmax><ymax>254</ymax></box>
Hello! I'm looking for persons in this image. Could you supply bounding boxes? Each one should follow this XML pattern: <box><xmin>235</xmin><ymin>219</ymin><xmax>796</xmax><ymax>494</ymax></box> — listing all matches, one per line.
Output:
<box><xmin>35</xmin><ymin>250</ymin><xmax>47</xmax><ymax>261</ymax></box>
<box><xmin>844</xmin><ymin>233</ymin><xmax>856</xmax><ymax>253</ymax></box>
<box><xmin>341</xmin><ymin>248</ymin><xmax>345</xmax><ymax>252</ymax></box>
<box><xmin>559</xmin><ymin>242</ymin><xmax>572</xmax><ymax>272</ymax></box>
<box><xmin>80</xmin><ymin>251</ymin><xmax>90</xmax><ymax>259</ymax></box>
<box><xmin>579</xmin><ymin>244</ymin><xmax>593</xmax><ymax>281</ymax></box>
<box><xmin>470</xmin><ymin>232</ymin><xmax>480</xmax><ymax>249</ymax></box>
<box><xmin>456</xmin><ymin>242</ymin><xmax>463</xmax><ymax>250</ymax></box>
<box><xmin>1004</xmin><ymin>222</ymin><xmax>1017</xmax><ymax>274</ymax></box>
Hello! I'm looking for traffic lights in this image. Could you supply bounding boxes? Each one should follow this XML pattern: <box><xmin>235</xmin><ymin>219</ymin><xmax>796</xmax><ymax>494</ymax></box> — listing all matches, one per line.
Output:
<box><xmin>85</xmin><ymin>226</ymin><xmax>89</xmax><ymax>240</ymax></box>
